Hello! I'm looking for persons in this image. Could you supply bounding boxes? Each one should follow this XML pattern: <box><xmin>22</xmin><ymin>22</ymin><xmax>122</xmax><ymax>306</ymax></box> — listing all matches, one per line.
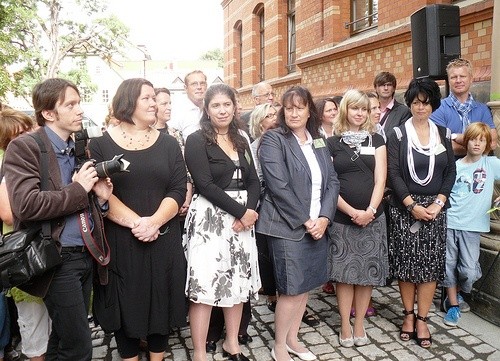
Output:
<box><xmin>387</xmin><ymin>76</ymin><xmax>457</xmax><ymax>348</ymax></box>
<box><xmin>326</xmin><ymin>90</ymin><xmax>389</xmax><ymax>348</ymax></box>
<box><xmin>0</xmin><ymin>103</ymin><xmax>53</xmax><ymax>361</ymax></box>
<box><xmin>4</xmin><ymin>78</ymin><xmax>113</xmax><ymax>361</ymax></box>
<box><xmin>443</xmin><ymin>122</ymin><xmax>500</xmax><ymax>327</ymax></box>
<box><xmin>373</xmin><ymin>72</ymin><xmax>412</xmax><ymax>134</ymax></box>
<box><xmin>429</xmin><ymin>58</ymin><xmax>497</xmax><ymax>161</ymax></box>
<box><xmin>150</xmin><ymin>70</ymin><xmax>386</xmax><ymax>353</ymax></box>
<box><xmin>89</xmin><ymin>78</ymin><xmax>187</xmax><ymax>361</ymax></box>
<box><xmin>184</xmin><ymin>83</ymin><xmax>261</xmax><ymax>361</ymax></box>
<box><xmin>256</xmin><ymin>85</ymin><xmax>340</xmax><ymax>361</ymax></box>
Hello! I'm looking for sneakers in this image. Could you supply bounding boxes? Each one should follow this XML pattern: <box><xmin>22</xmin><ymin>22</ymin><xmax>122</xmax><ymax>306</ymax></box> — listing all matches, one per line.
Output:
<box><xmin>443</xmin><ymin>306</ymin><xmax>462</xmax><ymax>326</ymax></box>
<box><xmin>444</xmin><ymin>293</ymin><xmax>457</xmax><ymax>311</ymax></box>
<box><xmin>457</xmin><ymin>293</ymin><xmax>470</xmax><ymax>313</ymax></box>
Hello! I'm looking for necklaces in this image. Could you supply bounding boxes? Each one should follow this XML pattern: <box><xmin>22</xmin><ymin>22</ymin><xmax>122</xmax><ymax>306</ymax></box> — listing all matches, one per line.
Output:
<box><xmin>212</xmin><ymin>128</ymin><xmax>229</xmax><ymax>141</ymax></box>
<box><xmin>120</xmin><ymin>124</ymin><xmax>151</xmax><ymax>149</ymax></box>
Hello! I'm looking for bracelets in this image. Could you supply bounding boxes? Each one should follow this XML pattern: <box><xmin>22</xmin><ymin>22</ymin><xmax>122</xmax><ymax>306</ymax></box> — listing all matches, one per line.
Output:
<box><xmin>324</xmin><ymin>217</ymin><xmax>331</xmax><ymax>225</ymax></box>
<box><xmin>434</xmin><ymin>199</ymin><xmax>444</xmax><ymax>207</ymax></box>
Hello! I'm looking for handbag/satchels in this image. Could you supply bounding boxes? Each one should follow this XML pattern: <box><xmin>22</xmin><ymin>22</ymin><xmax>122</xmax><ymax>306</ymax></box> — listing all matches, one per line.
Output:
<box><xmin>0</xmin><ymin>227</ymin><xmax>64</xmax><ymax>289</ymax></box>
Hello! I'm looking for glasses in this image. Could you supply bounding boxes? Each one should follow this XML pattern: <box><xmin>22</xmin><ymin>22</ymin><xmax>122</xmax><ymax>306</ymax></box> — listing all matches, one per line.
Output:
<box><xmin>266</xmin><ymin>113</ymin><xmax>277</xmax><ymax>118</ymax></box>
<box><xmin>258</xmin><ymin>92</ymin><xmax>276</xmax><ymax>97</ymax></box>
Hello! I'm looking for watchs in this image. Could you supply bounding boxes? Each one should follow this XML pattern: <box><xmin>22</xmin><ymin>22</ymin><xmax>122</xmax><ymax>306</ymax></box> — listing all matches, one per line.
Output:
<box><xmin>451</xmin><ymin>133</ymin><xmax>457</xmax><ymax>141</ymax></box>
<box><xmin>407</xmin><ymin>202</ymin><xmax>416</xmax><ymax>211</ymax></box>
<box><xmin>369</xmin><ymin>206</ymin><xmax>376</xmax><ymax>213</ymax></box>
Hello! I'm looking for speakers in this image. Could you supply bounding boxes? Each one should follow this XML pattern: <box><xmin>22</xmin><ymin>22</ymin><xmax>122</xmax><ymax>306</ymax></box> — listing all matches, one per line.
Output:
<box><xmin>410</xmin><ymin>4</ymin><xmax>461</xmax><ymax>80</ymax></box>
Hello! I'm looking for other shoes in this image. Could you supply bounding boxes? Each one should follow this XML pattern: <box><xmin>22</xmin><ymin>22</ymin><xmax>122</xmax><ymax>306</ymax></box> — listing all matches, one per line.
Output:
<box><xmin>268</xmin><ymin>300</ymin><xmax>277</xmax><ymax>312</ymax></box>
<box><xmin>366</xmin><ymin>307</ymin><xmax>373</xmax><ymax>315</ymax></box>
<box><xmin>350</xmin><ymin>308</ymin><xmax>356</xmax><ymax>316</ymax></box>
<box><xmin>323</xmin><ymin>284</ymin><xmax>335</xmax><ymax>293</ymax></box>
<box><xmin>302</xmin><ymin>310</ymin><xmax>320</xmax><ymax>326</ymax></box>
<box><xmin>5</xmin><ymin>346</ymin><xmax>19</xmax><ymax>359</ymax></box>
<box><xmin>286</xmin><ymin>343</ymin><xmax>317</xmax><ymax>361</ymax></box>
<box><xmin>355</xmin><ymin>325</ymin><xmax>368</xmax><ymax>346</ymax></box>
<box><xmin>271</xmin><ymin>348</ymin><xmax>294</xmax><ymax>361</ymax></box>
<box><xmin>339</xmin><ymin>324</ymin><xmax>354</xmax><ymax>347</ymax></box>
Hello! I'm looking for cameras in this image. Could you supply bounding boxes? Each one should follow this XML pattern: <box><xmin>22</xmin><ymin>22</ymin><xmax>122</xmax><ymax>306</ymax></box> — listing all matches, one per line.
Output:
<box><xmin>74</xmin><ymin>152</ymin><xmax>130</xmax><ymax>177</ymax></box>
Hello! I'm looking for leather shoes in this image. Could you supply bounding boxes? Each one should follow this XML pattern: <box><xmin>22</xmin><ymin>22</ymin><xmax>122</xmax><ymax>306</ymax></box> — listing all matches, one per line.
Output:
<box><xmin>205</xmin><ymin>342</ymin><xmax>217</xmax><ymax>353</ymax></box>
<box><xmin>238</xmin><ymin>334</ymin><xmax>253</xmax><ymax>344</ymax></box>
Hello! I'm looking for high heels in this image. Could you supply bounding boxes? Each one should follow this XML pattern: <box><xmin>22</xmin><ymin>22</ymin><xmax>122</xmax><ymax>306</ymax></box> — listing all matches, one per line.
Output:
<box><xmin>222</xmin><ymin>347</ymin><xmax>248</xmax><ymax>361</ymax></box>
<box><xmin>401</xmin><ymin>309</ymin><xmax>416</xmax><ymax>341</ymax></box>
<box><xmin>415</xmin><ymin>314</ymin><xmax>432</xmax><ymax>348</ymax></box>
